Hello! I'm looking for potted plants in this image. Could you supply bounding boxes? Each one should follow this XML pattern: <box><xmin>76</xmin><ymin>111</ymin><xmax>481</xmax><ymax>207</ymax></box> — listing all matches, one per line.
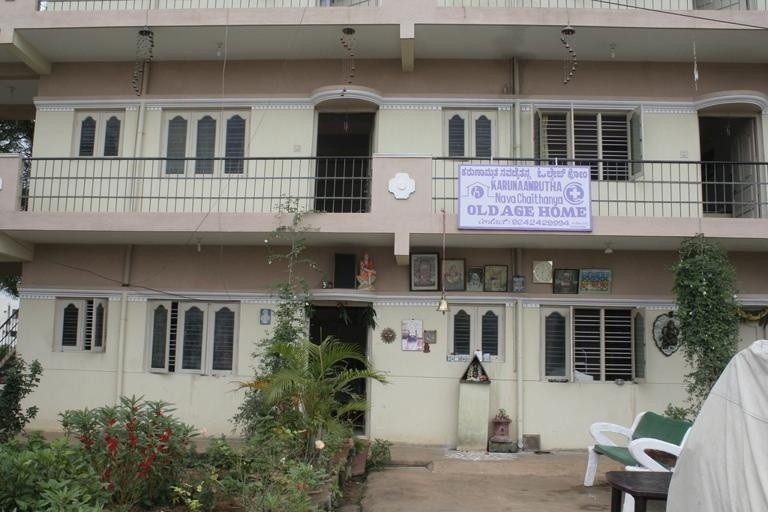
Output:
<box><xmin>73</xmin><ymin>192</ymin><xmax>394</xmax><ymax>510</ymax></box>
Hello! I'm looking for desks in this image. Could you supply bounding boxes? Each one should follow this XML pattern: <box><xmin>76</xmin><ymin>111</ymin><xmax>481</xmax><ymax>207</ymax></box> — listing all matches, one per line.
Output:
<box><xmin>604</xmin><ymin>467</ymin><xmax>675</xmax><ymax>511</ymax></box>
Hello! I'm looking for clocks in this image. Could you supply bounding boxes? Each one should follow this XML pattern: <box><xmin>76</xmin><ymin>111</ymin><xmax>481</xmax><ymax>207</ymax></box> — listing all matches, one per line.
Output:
<box><xmin>530</xmin><ymin>258</ymin><xmax>554</xmax><ymax>285</ymax></box>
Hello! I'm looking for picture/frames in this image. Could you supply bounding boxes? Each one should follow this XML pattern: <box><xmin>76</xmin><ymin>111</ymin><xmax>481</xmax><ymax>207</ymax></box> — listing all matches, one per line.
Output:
<box><xmin>409</xmin><ymin>250</ymin><xmax>529</xmax><ymax>294</ymax></box>
<box><xmin>550</xmin><ymin>268</ymin><xmax>613</xmax><ymax>294</ymax></box>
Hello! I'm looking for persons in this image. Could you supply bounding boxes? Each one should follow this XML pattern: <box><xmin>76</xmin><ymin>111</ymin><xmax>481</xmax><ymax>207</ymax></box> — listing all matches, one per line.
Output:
<box><xmin>401</xmin><ymin>323</ymin><xmax>422</xmax><ymax>342</ymax></box>
<box><xmin>355</xmin><ymin>250</ymin><xmax>377</xmax><ymax>285</ymax></box>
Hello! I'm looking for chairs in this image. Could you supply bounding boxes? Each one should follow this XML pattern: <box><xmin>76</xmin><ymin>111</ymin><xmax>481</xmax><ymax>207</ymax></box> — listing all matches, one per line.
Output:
<box><xmin>582</xmin><ymin>407</ymin><xmax>692</xmax><ymax>512</ymax></box>
